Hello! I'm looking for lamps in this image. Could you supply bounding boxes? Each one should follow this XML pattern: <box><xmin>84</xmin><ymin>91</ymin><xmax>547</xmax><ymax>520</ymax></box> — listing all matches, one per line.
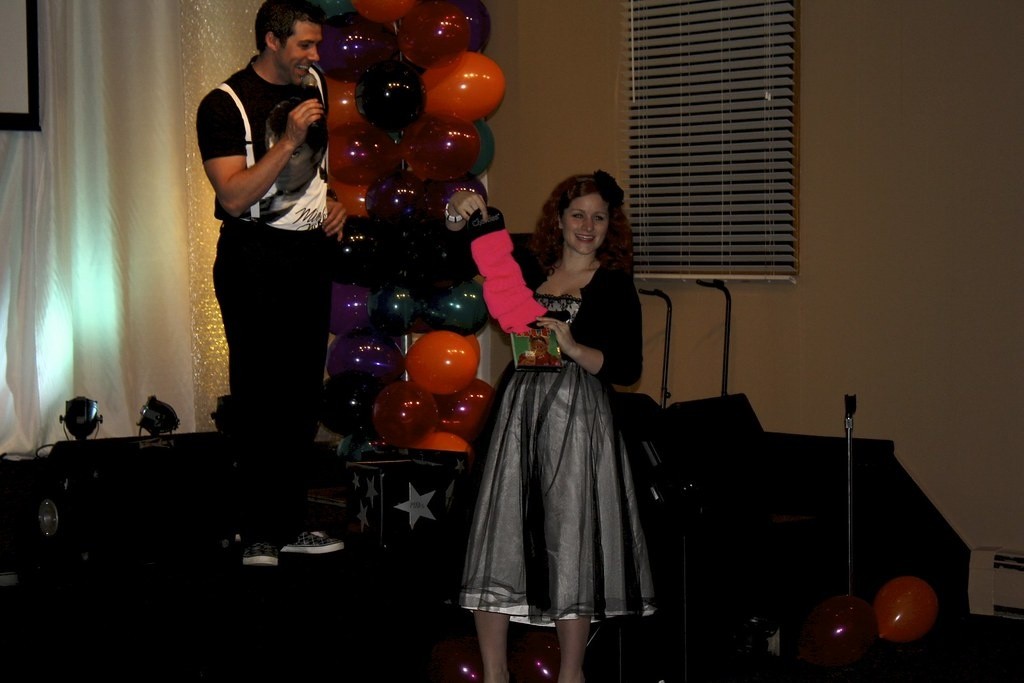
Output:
<box><xmin>61</xmin><ymin>394</ymin><xmax>105</xmax><ymax>442</ymax></box>
<box><xmin>210</xmin><ymin>395</ymin><xmax>232</xmax><ymax>434</ymax></box>
<box><xmin>131</xmin><ymin>393</ymin><xmax>179</xmax><ymax>440</ymax></box>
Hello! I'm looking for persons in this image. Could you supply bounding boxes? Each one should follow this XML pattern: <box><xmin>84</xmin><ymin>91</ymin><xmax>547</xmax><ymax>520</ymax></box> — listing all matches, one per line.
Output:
<box><xmin>440</xmin><ymin>168</ymin><xmax>645</xmax><ymax>682</ymax></box>
<box><xmin>197</xmin><ymin>1</ymin><xmax>347</xmax><ymax>570</ymax></box>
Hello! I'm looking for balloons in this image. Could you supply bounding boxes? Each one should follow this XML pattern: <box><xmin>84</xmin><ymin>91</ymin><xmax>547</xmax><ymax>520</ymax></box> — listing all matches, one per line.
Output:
<box><xmin>310</xmin><ymin>0</ymin><xmax>509</xmax><ymax>475</ymax></box>
<box><xmin>797</xmin><ymin>573</ymin><xmax>939</xmax><ymax>666</ymax></box>
<box><xmin>432</xmin><ymin>627</ymin><xmax>562</xmax><ymax>681</ymax></box>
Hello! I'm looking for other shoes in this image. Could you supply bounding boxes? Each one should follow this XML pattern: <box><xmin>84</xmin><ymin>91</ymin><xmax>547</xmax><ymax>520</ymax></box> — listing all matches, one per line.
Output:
<box><xmin>280</xmin><ymin>531</ymin><xmax>345</xmax><ymax>555</ymax></box>
<box><xmin>243</xmin><ymin>542</ymin><xmax>278</xmax><ymax>566</ymax></box>
